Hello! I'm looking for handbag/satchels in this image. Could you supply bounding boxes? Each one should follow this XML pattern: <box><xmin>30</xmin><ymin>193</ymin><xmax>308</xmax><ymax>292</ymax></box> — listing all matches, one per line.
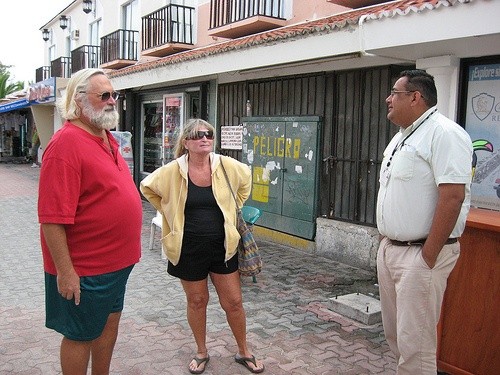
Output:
<box><xmin>236</xmin><ymin>209</ymin><xmax>262</xmax><ymax>276</ymax></box>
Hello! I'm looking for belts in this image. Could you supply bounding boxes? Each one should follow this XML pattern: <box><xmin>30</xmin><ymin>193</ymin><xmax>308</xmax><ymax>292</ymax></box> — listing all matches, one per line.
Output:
<box><xmin>389</xmin><ymin>238</ymin><xmax>457</xmax><ymax>246</ymax></box>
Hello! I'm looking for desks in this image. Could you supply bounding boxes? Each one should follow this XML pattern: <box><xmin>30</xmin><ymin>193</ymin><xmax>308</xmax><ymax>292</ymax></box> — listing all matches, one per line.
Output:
<box><xmin>431</xmin><ymin>208</ymin><xmax>500</xmax><ymax>375</ymax></box>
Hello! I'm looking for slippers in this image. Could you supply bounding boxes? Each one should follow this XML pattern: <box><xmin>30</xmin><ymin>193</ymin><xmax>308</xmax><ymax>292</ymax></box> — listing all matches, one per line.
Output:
<box><xmin>234</xmin><ymin>352</ymin><xmax>264</xmax><ymax>373</ymax></box>
<box><xmin>188</xmin><ymin>352</ymin><xmax>210</xmax><ymax>375</ymax></box>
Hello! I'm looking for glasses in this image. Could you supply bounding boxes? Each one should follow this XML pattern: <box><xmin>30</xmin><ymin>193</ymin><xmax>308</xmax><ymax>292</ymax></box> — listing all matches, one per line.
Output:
<box><xmin>186</xmin><ymin>130</ymin><xmax>214</xmax><ymax>140</ymax></box>
<box><xmin>390</xmin><ymin>89</ymin><xmax>424</xmax><ymax>99</ymax></box>
<box><xmin>80</xmin><ymin>90</ymin><xmax>120</xmax><ymax>101</ymax></box>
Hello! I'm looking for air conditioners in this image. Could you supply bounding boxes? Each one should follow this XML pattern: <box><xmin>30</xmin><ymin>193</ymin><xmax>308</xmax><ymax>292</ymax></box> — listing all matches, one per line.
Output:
<box><xmin>71</xmin><ymin>29</ymin><xmax>79</xmax><ymax>39</ymax></box>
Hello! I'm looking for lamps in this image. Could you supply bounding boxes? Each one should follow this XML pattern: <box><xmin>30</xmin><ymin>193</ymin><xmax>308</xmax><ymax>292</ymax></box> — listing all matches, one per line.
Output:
<box><xmin>59</xmin><ymin>15</ymin><xmax>71</xmax><ymax>31</ymax></box>
<box><xmin>42</xmin><ymin>28</ymin><xmax>52</xmax><ymax>42</ymax></box>
<box><xmin>82</xmin><ymin>0</ymin><xmax>95</xmax><ymax>14</ymax></box>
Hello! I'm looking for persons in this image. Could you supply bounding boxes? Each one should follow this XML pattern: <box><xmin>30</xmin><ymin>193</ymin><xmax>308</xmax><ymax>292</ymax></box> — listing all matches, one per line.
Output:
<box><xmin>31</xmin><ymin>127</ymin><xmax>41</xmax><ymax>167</ymax></box>
<box><xmin>140</xmin><ymin>118</ymin><xmax>264</xmax><ymax>374</ymax></box>
<box><xmin>38</xmin><ymin>68</ymin><xmax>142</xmax><ymax>375</ymax></box>
<box><xmin>376</xmin><ymin>69</ymin><xmax>474</xmax><ymax>375</ymax></box>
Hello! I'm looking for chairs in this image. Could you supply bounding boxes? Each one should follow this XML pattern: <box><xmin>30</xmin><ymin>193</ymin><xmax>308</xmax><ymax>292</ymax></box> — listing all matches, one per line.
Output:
<box><xmin>241</xmin><ymin>206</ymin><xmax>262</xmax><ymax>283</ymax></box>
<box><xmin>150</xmin><ymin>210</ymin><xmax>165</xmax><ymax>256</ymax></box>
